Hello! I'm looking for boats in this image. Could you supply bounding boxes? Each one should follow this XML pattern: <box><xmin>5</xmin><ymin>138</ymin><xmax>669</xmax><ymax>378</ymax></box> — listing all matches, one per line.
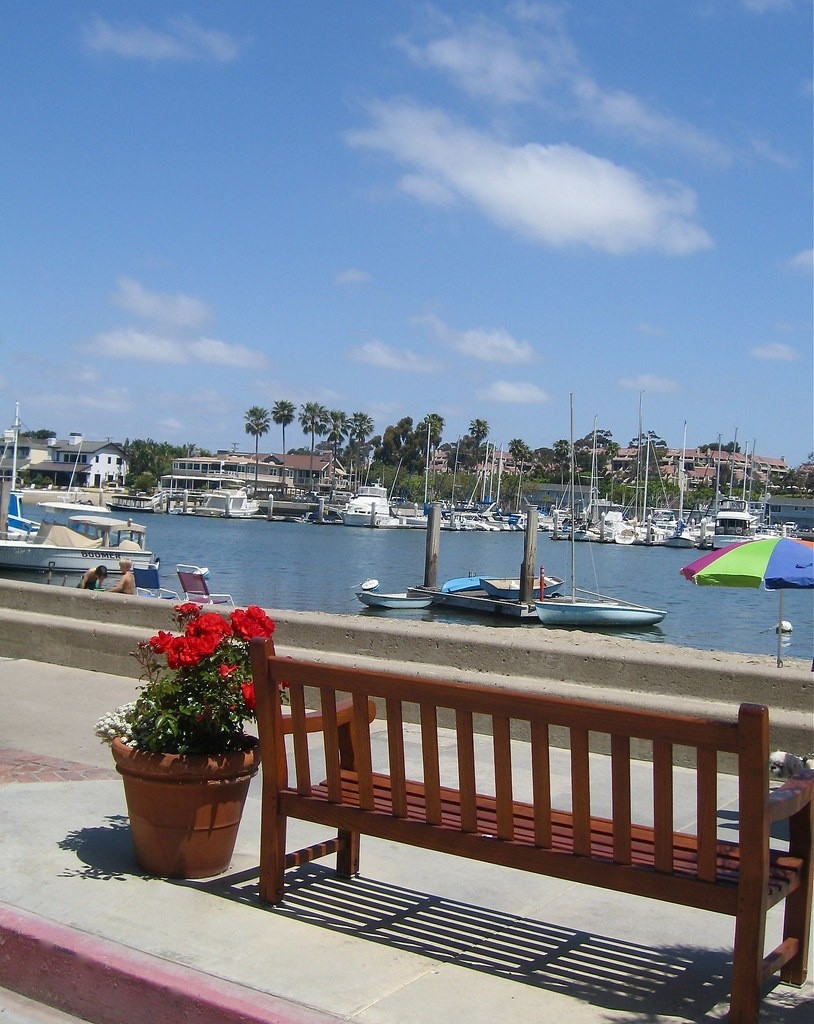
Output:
<box><xmin>38</xmin><ymin>501</ymin><xmax>111</xmax><ymax>520</ymax></box>
<box><xmin>0</xmin><ymin>398</ymin><xmax>160</xmax><ymax>575</ymax></box>
<box><xmin>341</xmin><ymin>476</ymin><xmax>407</xmax><ymax>528</ymax></box>
<box><xmin>104</xmin><ymin>494</ymin><xmax>154</xmax><ymax>513</ymax></box>
<box><xmin>193</xmin><ymin>488</ymin><xmax>261</xmax><ymax>518</ymax></box>
<box><xmin>358</xmin><ymin>591</ymin><xmax>436</xmax><ymax>609</ymax></box>
<box><xmin>478</xmin><ymin>575</ymin><xmax>565</xmax><ymax>600</ymax></box>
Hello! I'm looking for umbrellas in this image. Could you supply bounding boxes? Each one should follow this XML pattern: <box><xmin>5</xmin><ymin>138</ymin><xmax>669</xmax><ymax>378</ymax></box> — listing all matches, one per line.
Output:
<box><xmin>679</xmin><ymin>537</ymin><xmax>814</xmax><ymax>667</ymax></box>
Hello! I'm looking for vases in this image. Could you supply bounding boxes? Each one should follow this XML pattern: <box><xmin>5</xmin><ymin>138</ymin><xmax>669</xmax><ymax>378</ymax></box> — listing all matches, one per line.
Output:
<box><xmin>113</xmin><ymin>737</ymin><xmax>261</xmax><ymax>878</ymax></box>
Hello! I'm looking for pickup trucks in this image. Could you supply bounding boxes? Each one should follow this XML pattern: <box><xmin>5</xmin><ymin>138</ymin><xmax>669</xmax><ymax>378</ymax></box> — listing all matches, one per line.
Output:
<box><xmin>784</xmin><ymin>522</ymin><xmax>798</xmax><ymax>531</ymax></box>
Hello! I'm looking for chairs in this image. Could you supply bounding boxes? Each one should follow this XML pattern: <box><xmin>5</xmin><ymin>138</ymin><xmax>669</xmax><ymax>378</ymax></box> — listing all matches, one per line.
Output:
<box><xmin>133</xmin><ymin>562</ymin><xmax>180</xmax><ymax>599</ymax></box>
<box><xmin>176</xmin><ymin>564</ymin><xmax>235</xmax><ymax>606</ymax></box>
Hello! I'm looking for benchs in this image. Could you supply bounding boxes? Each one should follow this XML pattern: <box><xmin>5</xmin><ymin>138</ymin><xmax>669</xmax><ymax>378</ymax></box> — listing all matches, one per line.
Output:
<box><xmin>251</xmin><ymin>638</ymin><xmax>814</xmax><ymax>1024</ymax></box>
<box><xmin>526</xmin><ymin>789</ymin><xmax>537</xmax><ymax>796</ymax></box>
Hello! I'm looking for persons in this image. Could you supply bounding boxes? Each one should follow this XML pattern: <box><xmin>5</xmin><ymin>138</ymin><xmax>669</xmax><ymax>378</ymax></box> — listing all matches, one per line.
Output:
<box><xmin>148</xmin><ymin>489</ymin><xmax>155</xmax><ymax>497</ymax></box>
<box><xmin>76</xmin><ymin>565</ymin><xmax>107</xmax><ymax>590</ymax></box>
<box><xmin>549</xmin><ymin>503</ymin><xmax>556</xmax><ymax>517</ymax></box>
<box><xmin>775</xmin><ymin>522</ymin><xmax>778</xmax><ymax>530</ymax></box>
<box><xmin>110</xmin><ymin>559</ymin><xmax>136</xmax><ymax>595</ymax></box>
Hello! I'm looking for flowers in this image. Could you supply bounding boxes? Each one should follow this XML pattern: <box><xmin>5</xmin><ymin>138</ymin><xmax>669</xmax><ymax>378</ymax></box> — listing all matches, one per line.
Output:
<box><xmin>97</xmin><ymin>608</ymin><xmax>276</xmax><ymax>735</ymax></box>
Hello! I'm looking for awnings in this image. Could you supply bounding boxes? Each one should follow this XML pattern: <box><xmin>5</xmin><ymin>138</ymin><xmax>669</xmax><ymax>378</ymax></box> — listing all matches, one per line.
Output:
<box><xmin>25</xmin><ymin>461</ymin><xmax>92</xmax><ymax>473</ymax></box>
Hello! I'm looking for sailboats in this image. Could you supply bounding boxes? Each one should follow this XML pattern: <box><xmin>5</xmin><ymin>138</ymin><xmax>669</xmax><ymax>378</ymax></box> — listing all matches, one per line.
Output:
<box><xmin>409</xmin><ymin>391</ymin><xmax>799</xmax><ymax>550</ymax></box>
<box><xmin>407</xmin><ymin>392</ymin><xmax>670</xmax><ymax>626</ymax></box>
